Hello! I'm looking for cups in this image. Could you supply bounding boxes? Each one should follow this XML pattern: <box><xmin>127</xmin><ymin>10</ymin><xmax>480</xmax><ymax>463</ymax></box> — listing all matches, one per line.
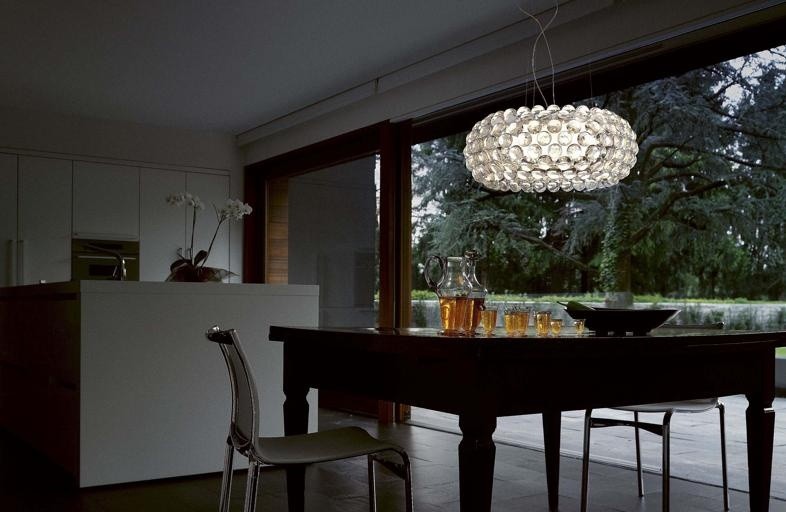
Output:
<box><xmin>573</xmin><ymin>317</ymin><xmax>585</xmax><ymax>334</ymax></box>
<box><xmin>517</xmin><ymin>307</ymin><xmax>532</xmax><ymax>336</ymax></box>
<box><xmin>549</xmin><ymin>319</ymin><xmax>564</xmax><ymax>333</ymax></box>
<box><xmin>534</xmin><ymin>311</ymin><xmax>552</xmax><ymax>335</ymax></box>
<box><xmin>480</xmin><ymin>306</ymin><xmax>499</xmax><ymax>335</ymax></box>
<box><xmin>504</xmin><ymin>306</ymin><xmax>518</xmax><ymax>335</ymax></box>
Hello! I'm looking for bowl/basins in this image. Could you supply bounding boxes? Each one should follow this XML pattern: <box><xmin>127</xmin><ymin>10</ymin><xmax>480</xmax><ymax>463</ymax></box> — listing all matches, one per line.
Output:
<box><xmin>563</xmin><ymin>306</ymin><xmax>682</xmax><ymax>333</ymax></box>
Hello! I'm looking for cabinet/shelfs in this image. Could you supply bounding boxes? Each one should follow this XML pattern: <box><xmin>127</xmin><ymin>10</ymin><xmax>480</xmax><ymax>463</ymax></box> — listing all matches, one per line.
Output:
<box><xmin>0</xmin><ymin>145</ymin><xmax>72</xmax><ymax>290</ymax></box>
<box><xmin>73</xmin><ymin>152</ymin><xmax>140</xmax><ymax>243</ymax></box>
<box><xmin>124</xmin><ymin>160</ymin><xmax>236</xmax><ymax>284</ymax></box>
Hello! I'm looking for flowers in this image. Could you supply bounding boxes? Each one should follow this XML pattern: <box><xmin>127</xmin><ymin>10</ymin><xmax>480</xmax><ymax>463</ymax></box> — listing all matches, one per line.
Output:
<box><xmin>164</xmin><ymin>188</ymin><xmax>254</xmax><ymax>272</ymax></box>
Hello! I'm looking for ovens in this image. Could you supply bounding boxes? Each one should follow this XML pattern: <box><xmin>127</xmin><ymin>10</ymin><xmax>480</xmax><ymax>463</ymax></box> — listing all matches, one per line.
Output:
<box><xmin>70</xmin><ymin>240</ymin><xmax>139</xmax><ymax>280</ymax></box>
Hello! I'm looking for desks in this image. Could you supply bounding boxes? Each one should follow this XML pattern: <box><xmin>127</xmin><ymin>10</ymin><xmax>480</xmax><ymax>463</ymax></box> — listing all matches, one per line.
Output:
<box><xmin>265</xmin><ymin>319</ymin><xmax>786</xmax><ymax>512</ymax></box>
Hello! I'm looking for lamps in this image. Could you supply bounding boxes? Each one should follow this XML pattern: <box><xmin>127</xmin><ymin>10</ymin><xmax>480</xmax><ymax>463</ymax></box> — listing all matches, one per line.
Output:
<box><xmin>458</xmin><ymin>0</ymin><xmax>642</xmax><ymax>204</ymax></box>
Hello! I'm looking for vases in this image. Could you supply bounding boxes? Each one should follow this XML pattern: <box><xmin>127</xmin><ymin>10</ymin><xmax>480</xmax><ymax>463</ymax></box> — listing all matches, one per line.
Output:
<box><xmin>161</xmin><ymin>262</ymin><xmax>242</xmax><ymax>286</ymax></box>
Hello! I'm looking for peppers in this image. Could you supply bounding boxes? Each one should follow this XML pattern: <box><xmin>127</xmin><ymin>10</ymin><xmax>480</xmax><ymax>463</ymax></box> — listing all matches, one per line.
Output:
<box><xmin>557</xmin><ymin>300</ymin><xmax>597</xmax><ymax>311</ymax></box>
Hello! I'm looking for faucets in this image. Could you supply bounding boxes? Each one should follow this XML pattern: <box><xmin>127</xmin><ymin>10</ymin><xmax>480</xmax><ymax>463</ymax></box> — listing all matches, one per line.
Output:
<box><xmin>80</xmin><ymin>242</ymin><xmax>128</xmax><ymax>281</ymax></box>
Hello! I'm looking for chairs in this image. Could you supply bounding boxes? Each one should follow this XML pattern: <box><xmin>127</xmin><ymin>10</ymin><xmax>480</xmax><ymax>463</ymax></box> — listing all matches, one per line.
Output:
<box><xmin>201</xmin><ymin>322</ymin><xmax>415</xmax><ymax>511</ymax></box>
<box><xmin>580</xmin><ymin>321</ymin><xmax>737</xmax><ymax>512</ymax></box>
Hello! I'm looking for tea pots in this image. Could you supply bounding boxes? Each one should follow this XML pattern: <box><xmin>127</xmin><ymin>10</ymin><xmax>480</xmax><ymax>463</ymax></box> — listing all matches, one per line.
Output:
<box><xmin>422</xmin><ymin>254</ymin><xmax>473</xmax><ymax>335</ymax></box>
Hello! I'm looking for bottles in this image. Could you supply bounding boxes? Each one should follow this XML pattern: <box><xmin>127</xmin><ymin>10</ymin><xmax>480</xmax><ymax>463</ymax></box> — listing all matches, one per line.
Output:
<box><xmin>462</xmin><ymin>249</ymin><xmax>488</xmax><ymax>335</ymax></box>
